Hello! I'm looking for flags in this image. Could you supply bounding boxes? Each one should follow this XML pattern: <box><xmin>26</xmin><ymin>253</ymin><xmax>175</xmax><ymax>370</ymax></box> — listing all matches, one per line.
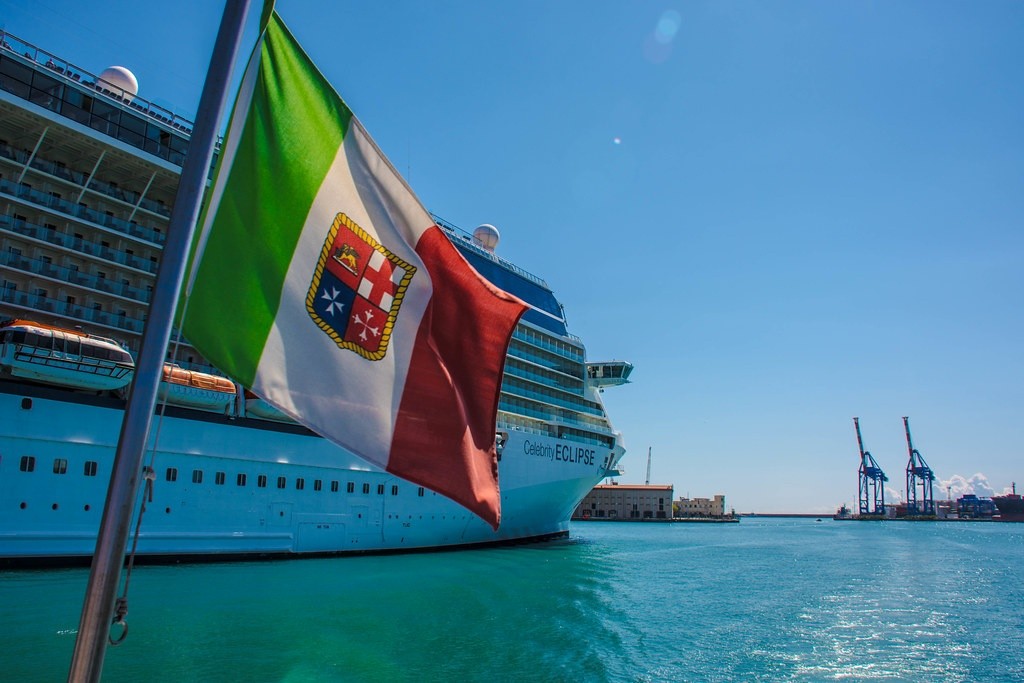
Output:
<box><xmin>172</xmin><ymin>0</ymin><xmax>530</xmax><ymax>531</ymax></box>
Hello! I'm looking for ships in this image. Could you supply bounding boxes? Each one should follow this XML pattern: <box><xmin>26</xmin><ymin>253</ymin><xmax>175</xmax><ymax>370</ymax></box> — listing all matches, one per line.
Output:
<box><xmin>0</xmin><ymin>27</ymin><xmax>626</xmax><ymax>579</ymax></box>
